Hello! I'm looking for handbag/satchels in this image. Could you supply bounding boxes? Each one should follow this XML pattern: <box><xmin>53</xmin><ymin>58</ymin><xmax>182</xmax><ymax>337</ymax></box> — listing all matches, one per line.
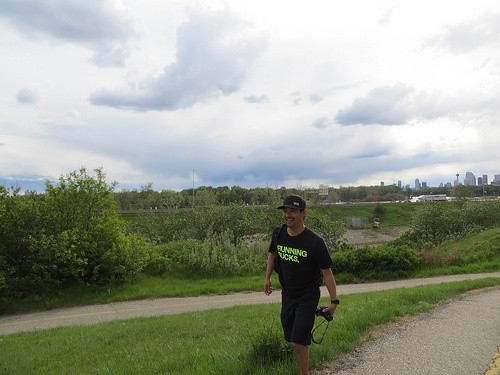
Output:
<box><xmin>273</xmin><ymin>223</ymin><xmax>286</xmax><ymax>273</ymax></box>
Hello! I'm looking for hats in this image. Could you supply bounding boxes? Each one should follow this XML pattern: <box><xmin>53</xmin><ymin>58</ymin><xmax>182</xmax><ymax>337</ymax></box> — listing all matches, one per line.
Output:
<box><xmin>277</xmin><ymin>195</ymin><xmax>306</xmax><ymax>219</ymax></box>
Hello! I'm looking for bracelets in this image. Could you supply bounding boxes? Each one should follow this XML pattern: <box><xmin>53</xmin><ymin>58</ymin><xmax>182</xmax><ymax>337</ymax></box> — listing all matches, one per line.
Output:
<box><xmin>330</xmin><ymin>300</ymin><xmax>339</xmax><ymax>304</ymax></box>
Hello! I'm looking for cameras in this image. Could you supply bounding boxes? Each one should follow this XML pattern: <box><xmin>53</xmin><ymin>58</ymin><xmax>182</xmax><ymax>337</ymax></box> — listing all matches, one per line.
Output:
<box><xmin>316</xmin><ymin>306</ymin><xmax>333</xmax><ymax>321</ymax></box>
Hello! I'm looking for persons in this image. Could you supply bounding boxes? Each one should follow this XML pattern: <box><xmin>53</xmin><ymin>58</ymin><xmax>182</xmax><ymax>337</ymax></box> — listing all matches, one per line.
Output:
<box><xmin>264</xmin><ymin>195</ymin><xmax>339</xmax><ymax>375</ymax></box>
